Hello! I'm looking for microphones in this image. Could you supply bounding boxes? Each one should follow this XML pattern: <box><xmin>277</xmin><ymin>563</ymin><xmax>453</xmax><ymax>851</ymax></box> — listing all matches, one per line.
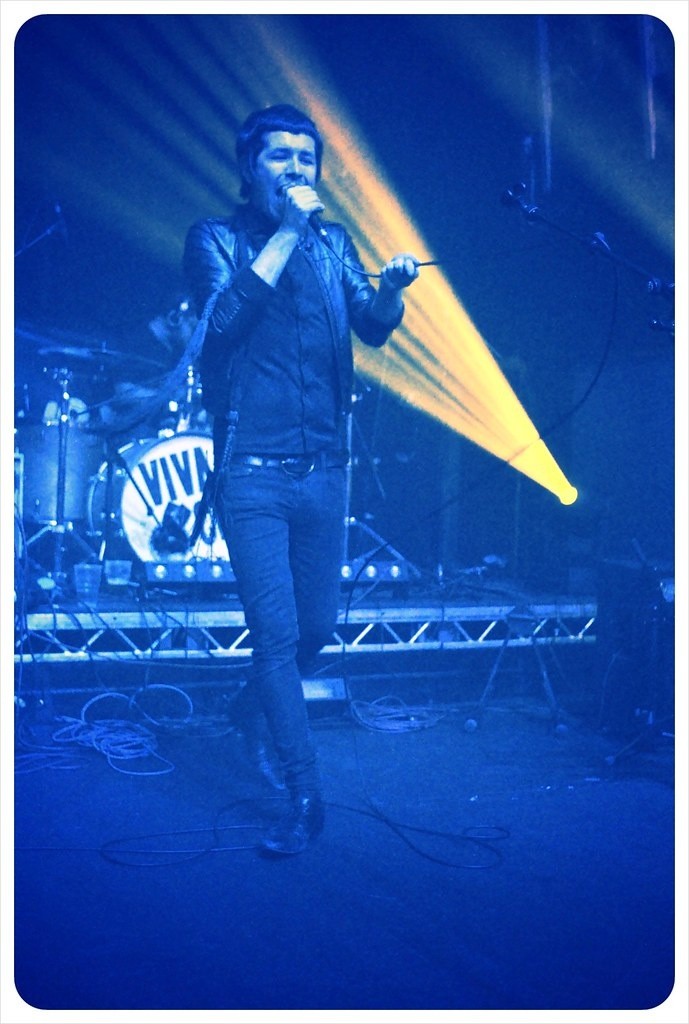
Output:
<box><xmin>281</xmin><ymin>182</ymin><xmax>332</xmax><ymax>249</ymax></box>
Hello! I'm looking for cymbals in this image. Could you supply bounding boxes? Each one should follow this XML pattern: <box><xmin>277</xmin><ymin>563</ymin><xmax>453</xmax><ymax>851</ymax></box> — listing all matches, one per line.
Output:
<box><xmin>38</xmin><ymin>343</ymin><xmax>170</xmax><ymax>374</ymax></box>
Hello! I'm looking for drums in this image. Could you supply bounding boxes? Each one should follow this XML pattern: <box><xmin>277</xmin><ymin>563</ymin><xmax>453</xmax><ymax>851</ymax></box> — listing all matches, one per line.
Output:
<box><xmin>15</xmin><ymin>424</ymin><xmax>101</xmax><ymax>522</ymax></box>
<box><xmin>87</xmin><ymin>431</ymin><xmax>231</xmax><ymax>572</ymax></box>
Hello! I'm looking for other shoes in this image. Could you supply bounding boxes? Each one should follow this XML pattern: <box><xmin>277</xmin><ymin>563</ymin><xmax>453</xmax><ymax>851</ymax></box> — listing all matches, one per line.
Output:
<box><xmin>229</xmin><ymin>696</ymin><xmax>285</xmax><ymax>789</ymax></box>
<box><xmin>262</xmin><ymin>796</ymin><xmax>327</xmax><ymax>855</ymax></box>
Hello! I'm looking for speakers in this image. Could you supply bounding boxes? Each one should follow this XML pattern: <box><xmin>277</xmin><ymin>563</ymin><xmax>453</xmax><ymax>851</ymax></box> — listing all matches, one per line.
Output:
<box><xmin>597</xmin><ymin>558</ymin><xmax>674</xmax><ymax>746</ymax></box>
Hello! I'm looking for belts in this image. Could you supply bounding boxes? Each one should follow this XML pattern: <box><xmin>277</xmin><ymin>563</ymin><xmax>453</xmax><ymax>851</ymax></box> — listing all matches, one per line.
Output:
<box><xmin>229</xmin><ymin>452</ymin><xmax>349</xmax><ymax>479</ymax></box>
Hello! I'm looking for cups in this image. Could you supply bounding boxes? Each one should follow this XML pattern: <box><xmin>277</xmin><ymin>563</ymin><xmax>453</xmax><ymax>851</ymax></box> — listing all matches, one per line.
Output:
<box><xmin>105</xmin><ymin>559</ymin><xmax>132</xmax><ymax>586</ymax></box>
<box><xmin>74</xmin><ymin>564</ymin><xmax>103</xmax><ymax>607</ymax></box>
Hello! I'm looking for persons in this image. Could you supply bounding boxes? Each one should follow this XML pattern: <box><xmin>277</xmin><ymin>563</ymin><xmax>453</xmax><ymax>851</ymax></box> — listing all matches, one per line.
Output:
<box><xmin>186</xmin><ymin>104</ymin><xmax>422</xmax><ymax>857</ymax></box>
<box><xmin>91</xmin><ymin>296</ymin><xmax>200</xmax><ymax>425</ymax></box>
